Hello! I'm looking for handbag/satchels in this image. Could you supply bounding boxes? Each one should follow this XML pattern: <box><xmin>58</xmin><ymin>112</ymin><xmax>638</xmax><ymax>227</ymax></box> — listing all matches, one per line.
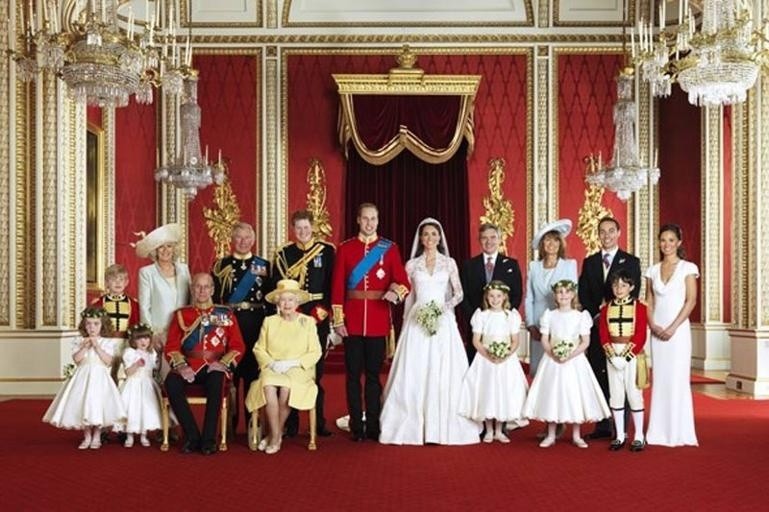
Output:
<box><xmin>246</xmin><ymin>414</ymin><xmax>263</xmax><ymax>451</ymax></box>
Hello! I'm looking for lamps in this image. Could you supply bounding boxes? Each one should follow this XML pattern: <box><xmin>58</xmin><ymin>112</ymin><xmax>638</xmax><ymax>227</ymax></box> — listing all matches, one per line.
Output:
<box><xmin>657</xmin><ymin>0</ymin><xmax>769</xmax><ymax>110</ymax></box>
<box><xmin>120</xmin><ymin>0</ymin><xmax>195</xmax><ymax>108</ymax></box>
<box><xmin>580</xmin><ymin>0</ymin><xmax>664</xmax><ymax>204</ymax></box>
<box><xmin>628</xmin><ymin>0</ymin><xmax>699</xmax><ymax>99</ymax></box>
<box><xmin>13</xmin><ymin>1</ymin><xmax>190</xmax><ymax>119</ymax></box>
<box><xmin>151</xmin><ymin>0</ymin><xmax>227</xmax><ymax>202</ymax></box>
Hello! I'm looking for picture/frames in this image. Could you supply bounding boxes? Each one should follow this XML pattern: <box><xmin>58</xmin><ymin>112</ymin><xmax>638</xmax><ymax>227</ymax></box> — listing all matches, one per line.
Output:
<box><xmin>82</xmin><ymin>118</ymin><xmax>109</xmax><ymax>294</ymax></box>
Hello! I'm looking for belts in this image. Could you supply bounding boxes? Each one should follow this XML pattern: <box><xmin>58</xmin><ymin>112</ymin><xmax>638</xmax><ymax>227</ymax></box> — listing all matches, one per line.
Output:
<box><xmin>310</xmin><ymin>292</ymin><xmax>324</xmax><ymax>303</ymax></box>
<box><xmin>225</xmin><ymin>301</ymin><xmax>264</xmax><ymax>311</ymax></box>
<box><xmin>183</xmin><ymin>350</ymin><xmax>224</xmax><ymax>360</ymax></box>
<box><xmin>344</xmin><ymin>290</ymin><xmax>386</xmax><ymax>300</ymax></box>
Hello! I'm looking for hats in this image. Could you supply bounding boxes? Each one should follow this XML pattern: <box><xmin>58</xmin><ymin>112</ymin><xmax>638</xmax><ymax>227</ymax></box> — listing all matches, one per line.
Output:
<box><xmin>484</xmin><ymin>257</ymin><xmax>495</xmax><ymax>284</ymax></box>
<box><xmin>530</xmin><ymin>218</ymin><xmax>573</xmax><ymax>251</ymax></box>
<box><xmin>132</xmin><ymin>222</ymin><xmax>184</xmax><ymax>261</ymax></box>
<box><xmin>264</xmin><ymin>278</ymin><xmax>311</xmax><ymax>306</ymax></box>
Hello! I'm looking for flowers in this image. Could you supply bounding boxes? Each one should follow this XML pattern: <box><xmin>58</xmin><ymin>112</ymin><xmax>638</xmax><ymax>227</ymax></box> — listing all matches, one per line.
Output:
<box><xmin>550</xmin><ymin>340</ymin><xmax>576</xmax><ymax>362</ymax></box>
<box><xmin>482</xmin><ymin>283</ymin><xmax>512</xmax><ymax>292</ymax></box>
<box><xmin>126</xmin><ymin>322</ymin><xmax>155</xmax><ymax>336</ymax></box>
<box><xmin>80</xmin><ymin>308</ymin><xmax>108</xmax><ymax>318</ymax></box>
<box><xmin>487</xmin><ymin>341</ymin><xmax>513</xmax><ymax>359</ymax></box>
<box><xmin>550</xmin><ymin>282</ymin><xmax>579</xmax><ymax>290</ymax></box>
<box><xmin>413</xmin><ymin>298</ymin><xmax>444</xmax><ymax>338</ymax></box>
<box><xmin>61</xmin><ymin>360</ymin><xmax>79</xmax><ymax>379</ymax></box>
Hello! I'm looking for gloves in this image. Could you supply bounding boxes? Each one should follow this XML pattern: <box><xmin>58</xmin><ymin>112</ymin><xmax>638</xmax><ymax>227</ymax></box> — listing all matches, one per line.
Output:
<box><xmin>268</xmin><ymin>359</ymin><xmax>300</xmax><ymax>374</ymax></box>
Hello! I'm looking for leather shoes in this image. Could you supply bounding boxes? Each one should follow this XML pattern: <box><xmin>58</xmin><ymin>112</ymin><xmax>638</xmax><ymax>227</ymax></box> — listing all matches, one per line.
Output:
<box><xmin>609</xmin><ymin>434</ymin><xmax>629</xmax><ymax>450</ymax></box>
<box><xmin>76</xmin><ymin>432</ymin><xmax>112</xmax><ymax>449</ymax></box>
<box><xmin>584</xmin><ymin>430</ymin><xmax>612</xmax><ymax>440</ymax></box>
<box><xmin>314</xmin><ymin>423</ymin><xmax>333</xmax><ymax>436</ymax></box>
<box><xmin>630</xmin><ymin>436</ymin><xmax>646</xmax><ymax>451</ymax></box>
<box><xmin>118</xmin><ymin>432</ymin><xmax>135</xmax><ymax>448</ymax></box>
<box><xmin>283</xmin><ymin>428</ymin><xmax>298</xmax><ymax>440</ymax></box>
<box><xmin>538</xmin><ymin>437</ymin><xmax>556</xmax><ymax>448</ymax></box>
<box><xmin>258</xmin><ymin>424</ymin><xmax>283</xmax><ymax>456</ymax></box>
<box><xmin>181</xmin><ymin>438</ymin><xmax>201</xmax><ymax>454</ymax></box>
<box><xmin>571</xmin><ymin>437</ymin><xmax>589</xmax><ymax>449</ymax></box>
<box><xmin>351</xmin><ymin>429</ymin><xmax>380</xmax><ymax>443</ymax></box>
<box><xmin>154</xmin><ymin>429</ymin><xmax>179</xmax><ymax>447</ymax></box>
<box><xmin>483</xmin><ymin>430</ymin><xmax>494</xmax><ymax>443</ymax></box>
<box><xmin>139</xmin><ymin>436</ymin><xmax>152</xmax><ymax>447</ymax></box>
<box><xmin>201</xmin><ymin>439</ymin><xmax>218</xmax><ymax>455</ymax></box>
<box><xmin>495</xmin><ymin>433</ymin><xmax>512</xmax><ymax>443</ymax></box>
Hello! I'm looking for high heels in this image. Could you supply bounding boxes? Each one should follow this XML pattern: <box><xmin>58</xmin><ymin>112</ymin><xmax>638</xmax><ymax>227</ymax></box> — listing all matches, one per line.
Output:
<box><xmin>536</xmin><ymin>422</ymin><xmax>567</xmax><ymax>439</ymax></box>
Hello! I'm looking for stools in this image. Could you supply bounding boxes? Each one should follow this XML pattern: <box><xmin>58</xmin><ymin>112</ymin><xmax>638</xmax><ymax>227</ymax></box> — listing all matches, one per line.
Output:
<box><xmin>248</xmin><ymin>395</ymin><xmax>319</xmax><ymax>452</ymax></box>
<box><xmin>156</xmin><ymin>366</ymin><xmax>235</xmax><ymax>455</ymax></box>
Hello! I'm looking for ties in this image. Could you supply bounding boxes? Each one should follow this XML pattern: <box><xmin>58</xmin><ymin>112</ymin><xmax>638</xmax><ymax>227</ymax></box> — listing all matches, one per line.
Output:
<box><xmin>602</xmin><ymin>254</ymin><xmax>610</xmax><ymax>270</ymax></box>
